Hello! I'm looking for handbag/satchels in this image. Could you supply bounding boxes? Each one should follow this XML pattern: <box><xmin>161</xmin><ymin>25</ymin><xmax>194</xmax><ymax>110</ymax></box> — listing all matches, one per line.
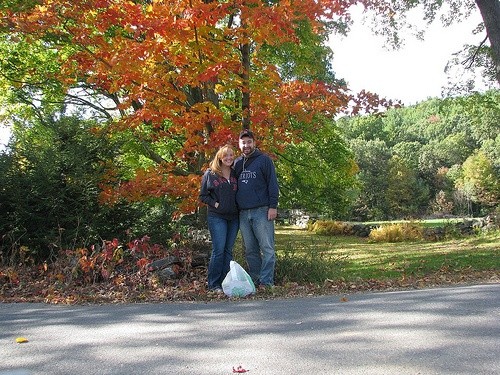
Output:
<box><xmin>221</xmin><ymin>260</ymin><xmax>255</xmax><ymax>298</ymax></box>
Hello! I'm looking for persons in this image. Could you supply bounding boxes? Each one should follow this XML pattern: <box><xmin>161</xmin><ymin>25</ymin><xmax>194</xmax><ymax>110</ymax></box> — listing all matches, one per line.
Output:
<box><xmin>199</xmin><ymin>145</ymin><xmax>241</xmax><ymax>293</ymax></box>
<box><xmin>232</xmin><ymin>130</ymin><xmax>279</xmax><ymax>289</ymax></box>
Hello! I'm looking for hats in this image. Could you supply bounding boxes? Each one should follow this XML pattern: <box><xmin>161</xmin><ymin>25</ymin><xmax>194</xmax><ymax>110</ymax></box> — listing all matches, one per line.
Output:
<box><xmin>239</xmin><ymin>129</ymin><xmax>254</xmax><ymax>140</ymax></box>
